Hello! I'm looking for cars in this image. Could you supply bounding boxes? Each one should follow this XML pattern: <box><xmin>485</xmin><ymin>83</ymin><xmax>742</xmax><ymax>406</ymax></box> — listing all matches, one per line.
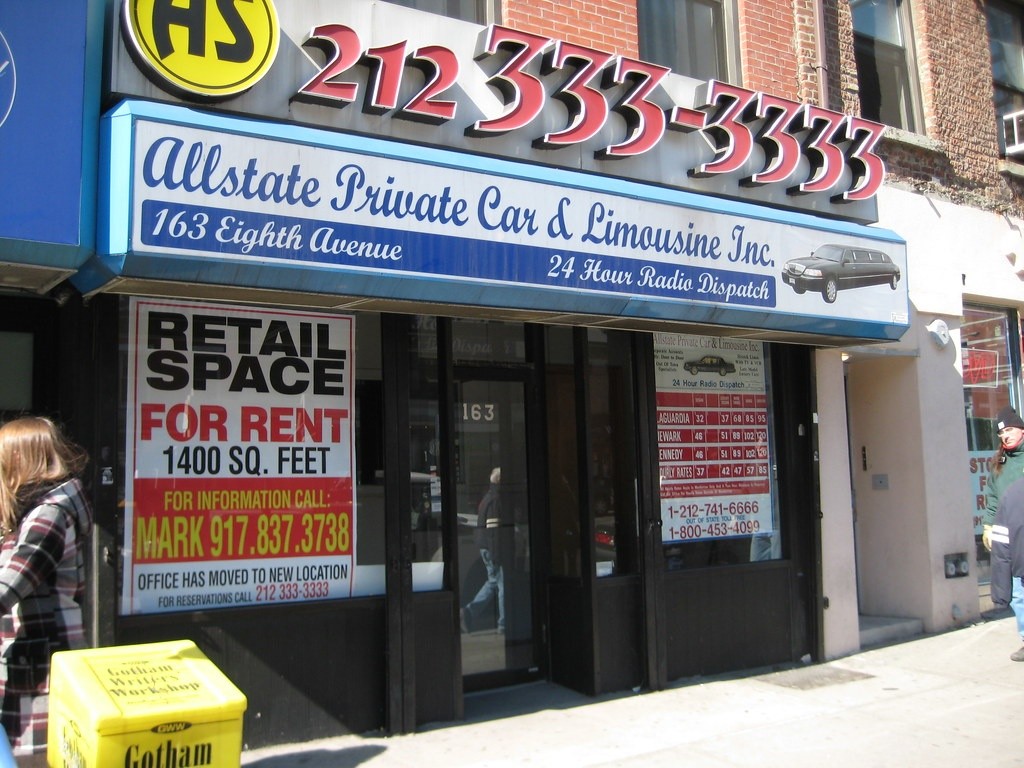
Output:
<box><xmin>781</xmin><ymin>244</ymin><xmax>900</xmax><ymax>304</ymax></box>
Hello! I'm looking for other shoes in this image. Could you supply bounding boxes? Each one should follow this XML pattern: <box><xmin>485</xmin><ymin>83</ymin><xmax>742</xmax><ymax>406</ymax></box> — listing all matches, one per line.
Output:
<box><xmin>497</xmin><ymin>629</ymin><xmax>504</xmax><ymax>634</ymax></box>
<box><xmin>460</xmin><ymin>608</ymin><xmax>473</xmax><ymax>634</ymax></box>
<box><xmin>1010</xmin><ymin>647</ymin><xmax>1024</xmax><ymax>661</ymax></box>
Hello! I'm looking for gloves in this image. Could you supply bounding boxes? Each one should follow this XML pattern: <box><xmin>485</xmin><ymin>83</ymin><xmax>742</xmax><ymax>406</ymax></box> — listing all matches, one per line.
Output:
<box><xmin>982</xmin><ymin>525</ymin><xmax>992</xmax><ymax>551</ymax></box>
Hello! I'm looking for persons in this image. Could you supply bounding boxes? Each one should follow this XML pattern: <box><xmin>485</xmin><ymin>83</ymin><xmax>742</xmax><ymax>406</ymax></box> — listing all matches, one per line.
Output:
<box><xmin>0</xmin><ymin>414</ymin><xmax>93</xmax><ymax>768</ymax></box>
<box><xmin>459</xmin><ymin>467</ymin><xmax>506</xmax><ymax>635</ymax></box>
<box><xmin>983</xmin><ymin>405</ymin><xmax>1024</xmax><ymax>662</ymax></box>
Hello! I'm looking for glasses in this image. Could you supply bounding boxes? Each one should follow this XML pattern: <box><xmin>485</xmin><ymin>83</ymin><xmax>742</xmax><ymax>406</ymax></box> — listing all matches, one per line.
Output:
<box><xmin>998</xmin><ymin>427</ymin><xmax>1014</xmax><ymax>437</ymax></box>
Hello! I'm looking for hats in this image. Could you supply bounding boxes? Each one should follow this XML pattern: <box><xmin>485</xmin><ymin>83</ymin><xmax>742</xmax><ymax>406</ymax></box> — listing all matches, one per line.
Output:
<box><xmin>995</xmin><ymin>406</ymin><xmax>1024</xmax><ymax>433</ymax></box>
